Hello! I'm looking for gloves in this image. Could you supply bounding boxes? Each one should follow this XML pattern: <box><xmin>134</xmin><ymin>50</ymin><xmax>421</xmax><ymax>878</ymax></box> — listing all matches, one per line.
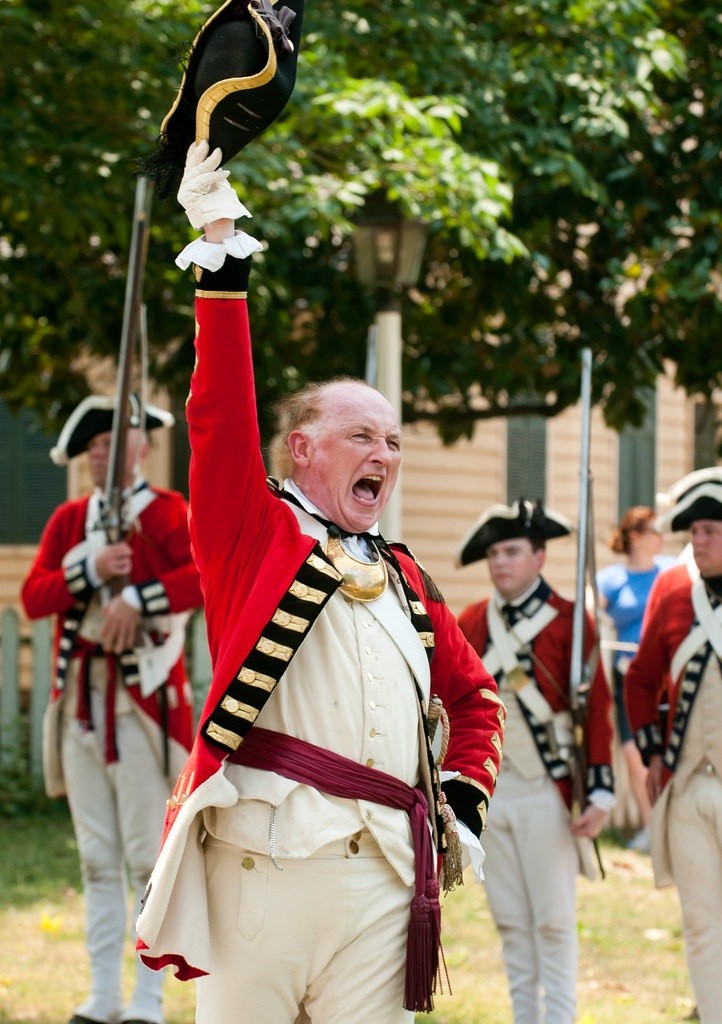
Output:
<box><xmin>177</xmin><ymin>138</ymin><xmax>253</xmax><ymax>231</ymax></box>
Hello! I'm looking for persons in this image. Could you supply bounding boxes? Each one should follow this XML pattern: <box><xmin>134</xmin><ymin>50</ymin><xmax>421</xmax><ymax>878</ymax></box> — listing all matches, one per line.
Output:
<box><xmin>585</xmin><ymin>505</ymin><xmax>678</xmax><ymax>851</ymax></box>
<box><xmin>455</xmin><ymin>496</ymin><xmax>616</xmax><ymax>1024</ymax></box>
<box><xmin>132</xmin><ymin>141</ymin><xmax>509</xmax><ymax>1024</ymax></box>
<box><xmin>20</xmin><ymin>397</ymin><xmax>205</xmax><ymax>1024</ymax></box>
<box><xmin>623</xmin><ymin>466</ymin><xmax>722</xmax><ymax>1024</ymax></box>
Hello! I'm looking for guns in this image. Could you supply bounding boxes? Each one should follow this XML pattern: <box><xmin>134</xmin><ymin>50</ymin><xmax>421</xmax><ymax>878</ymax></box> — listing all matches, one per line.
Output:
<box><xmin>93</xmin><ymin>172</ymin><xmax>156</xmax><ymax>649</ymax></box>
<box><xmin>568</xmin><ymin>348</ymin><xmax>596</xmax><ymax>823</ymax></box>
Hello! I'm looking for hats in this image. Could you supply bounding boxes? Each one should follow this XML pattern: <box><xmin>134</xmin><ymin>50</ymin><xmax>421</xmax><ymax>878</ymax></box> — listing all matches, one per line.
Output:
<box><xmin>138</xmin><ymin>0</ymin><xmax>304</xmax><ymax>197</ymax></box>
<box><xmin>666</xmin><ymin>466</ymin><xmax>722</xmax><ymax>530</ymax></box>
<box><xmin>453</xmin><ymin>496</ymin><xmax>573</xmax><ymax>568</ymax></box>
<box><xmin>51</xmin><ymin>395</ymin><xmax>174</xmax><ymax>466</ymax></box>
